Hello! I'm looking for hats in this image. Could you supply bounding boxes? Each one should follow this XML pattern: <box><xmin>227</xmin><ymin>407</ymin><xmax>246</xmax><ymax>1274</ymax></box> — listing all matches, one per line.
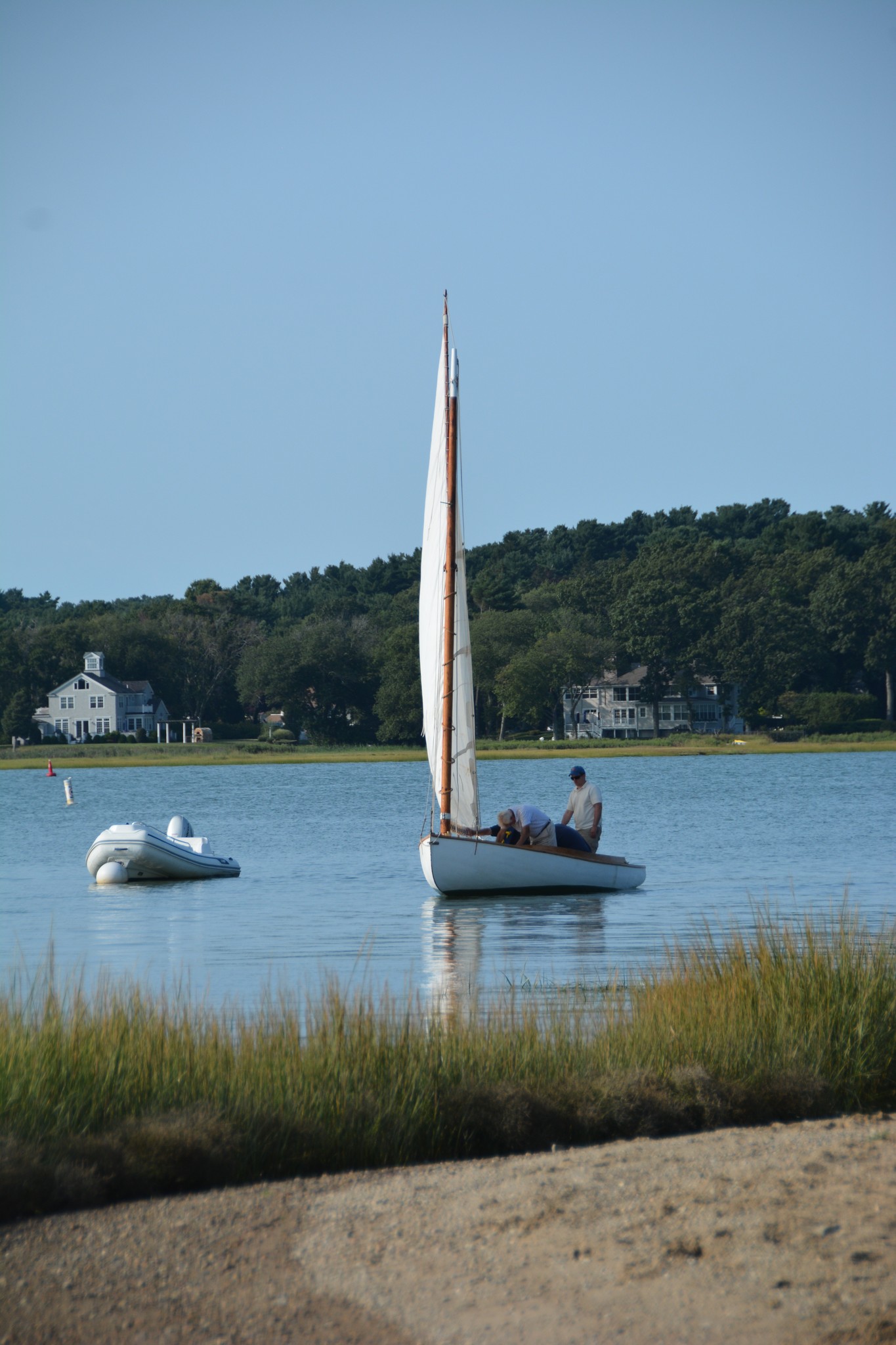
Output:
<box><xmin>568</xmin><ymin>766</ymin><xmax>585</xmax><ymax>777</ymax></box>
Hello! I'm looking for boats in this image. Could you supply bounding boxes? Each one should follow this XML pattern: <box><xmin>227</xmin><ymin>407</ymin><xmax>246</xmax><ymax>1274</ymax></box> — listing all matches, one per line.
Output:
<box><xmin>85</xmin><ymin>815</ymin><xmax>242</xmax><ymax>885</ymax></box>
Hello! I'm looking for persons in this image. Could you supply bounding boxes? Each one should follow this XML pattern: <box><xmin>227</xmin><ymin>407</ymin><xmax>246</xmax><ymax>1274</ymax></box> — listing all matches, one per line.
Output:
<box><xmin>463</xmin><ymin>804</ymin><xmax>594</xmax><ymax>853</ymax></box>
<box><xmin>561</xmin><ymin>765</ymin><xmax>603</xmax><ymax>852</ymax></box>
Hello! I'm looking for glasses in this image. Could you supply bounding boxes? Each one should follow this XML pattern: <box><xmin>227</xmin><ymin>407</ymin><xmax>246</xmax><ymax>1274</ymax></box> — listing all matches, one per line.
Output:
<box><xmin>571</xmin><ymin>774</ymin><xmax>583</xmax><ymax>780</ymax></box>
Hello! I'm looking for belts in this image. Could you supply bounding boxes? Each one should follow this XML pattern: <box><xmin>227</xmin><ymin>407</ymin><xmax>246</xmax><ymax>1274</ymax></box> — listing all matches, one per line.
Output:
<box><xmin>534</xmin><ymin>819</ymin><xmax>551</xmax><ymax>838</ymax></box>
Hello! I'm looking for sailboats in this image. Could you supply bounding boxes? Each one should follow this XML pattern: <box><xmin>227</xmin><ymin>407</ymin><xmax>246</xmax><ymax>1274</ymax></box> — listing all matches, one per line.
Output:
<box><xmin>417</xmin><ymin>299</ymin><xmax>646</xmax><ymax>899</ymax></box>
